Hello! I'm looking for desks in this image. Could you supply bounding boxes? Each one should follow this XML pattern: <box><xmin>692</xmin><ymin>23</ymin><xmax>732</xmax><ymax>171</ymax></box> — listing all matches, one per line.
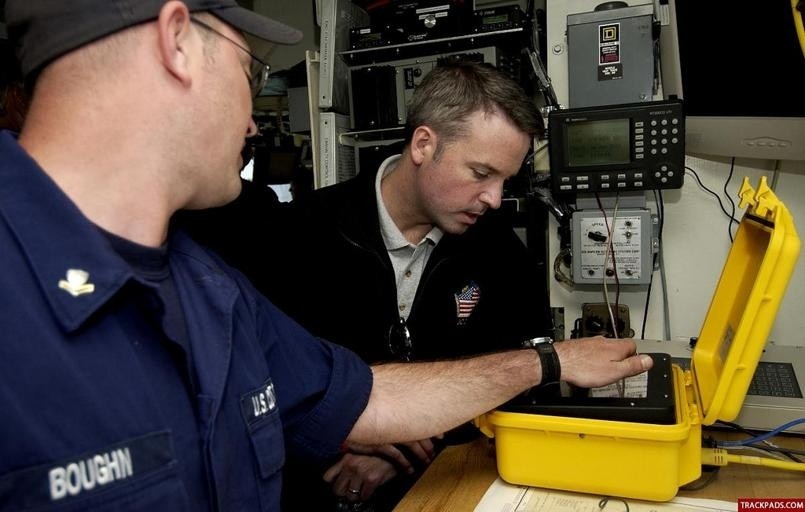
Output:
<box><xmin>384</xmin><ymin>419</ymin><xmax>805</xmax><ymax>512</ymax></box>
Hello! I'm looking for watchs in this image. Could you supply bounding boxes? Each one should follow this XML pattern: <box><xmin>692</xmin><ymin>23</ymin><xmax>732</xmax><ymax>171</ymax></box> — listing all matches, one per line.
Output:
<box><xmin>518</xmin><ymin>333</ymin><xmax>564</xmax><ymax>388</ymax></box>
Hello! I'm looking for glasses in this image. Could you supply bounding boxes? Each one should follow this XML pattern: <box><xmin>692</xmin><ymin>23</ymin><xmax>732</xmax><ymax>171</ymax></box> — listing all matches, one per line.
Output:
<box><xmin>189</xmin><ymin>15</ymin><xmax>270</xmax><ymax>98</ymax></box>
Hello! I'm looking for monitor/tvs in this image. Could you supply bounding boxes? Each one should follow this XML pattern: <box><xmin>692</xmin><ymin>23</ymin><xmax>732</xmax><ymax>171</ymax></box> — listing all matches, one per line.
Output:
<box><xmin>654</xmin><ymin>0</ymin><xmax>804</xmax><ymax>162</ymax></box>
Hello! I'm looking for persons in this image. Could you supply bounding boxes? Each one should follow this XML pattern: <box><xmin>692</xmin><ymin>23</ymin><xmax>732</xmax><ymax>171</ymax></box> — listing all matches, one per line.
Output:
<box><xmin>0</xmin><ymin>0</ymin><xmax>656</xmax><ymax>510</ymax></box>
<box><xmin>241</xmin><ymin>59</ymin><xmax>543</xmax><ymax>510</ymax></box>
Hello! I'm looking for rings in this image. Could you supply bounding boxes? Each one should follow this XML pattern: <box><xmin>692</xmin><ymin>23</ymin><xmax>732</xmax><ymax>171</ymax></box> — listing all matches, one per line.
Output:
<box><xmin>348</xmin><ymin>485</ymin><xmax>361</xmax><ymax>498</ymax></box>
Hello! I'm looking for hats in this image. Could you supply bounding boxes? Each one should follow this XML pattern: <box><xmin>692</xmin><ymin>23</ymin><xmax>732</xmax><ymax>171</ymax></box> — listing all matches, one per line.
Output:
<box><xmin>4</xmin><ymin>0</ymin><xmax>305</xmax><ymax>86</ymax></box>
